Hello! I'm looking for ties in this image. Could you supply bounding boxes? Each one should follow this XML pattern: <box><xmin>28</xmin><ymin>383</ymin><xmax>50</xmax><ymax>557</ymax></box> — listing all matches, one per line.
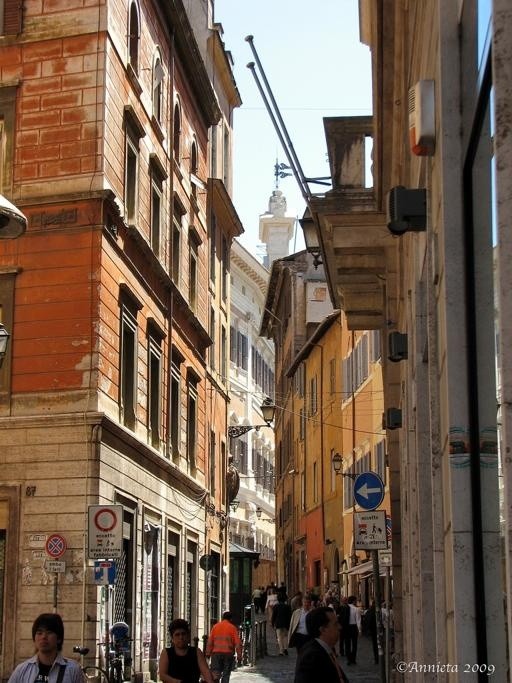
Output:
<box><xmin>331</xmin><ymin>651</ymin><xmax>344</xmax><ymax>683</ymax></box>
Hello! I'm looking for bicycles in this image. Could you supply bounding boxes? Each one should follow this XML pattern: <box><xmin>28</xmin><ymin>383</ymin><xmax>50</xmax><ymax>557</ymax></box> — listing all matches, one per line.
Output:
<box><xmin>73</xmin><ymin>638</ymin><xmax>133</xmax><ymax>683</ymax></box>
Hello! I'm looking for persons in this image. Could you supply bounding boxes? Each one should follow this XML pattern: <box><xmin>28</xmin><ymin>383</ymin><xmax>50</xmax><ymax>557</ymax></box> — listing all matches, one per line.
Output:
<box><xmin>294</xmin><ymin>607</ymin><xmax>351</xmax><ymax>683</ymax></box>
<box><xmin>200</xmin><ymin>667</ymin><xmax>222</xmax><ymax>683</ymax></box>
<box><xmin>252</xmin><ymin>581</ymin><xmax>395</xmax><ymax>666</ymax></box>
<box><xmin>159</xmin><ymin>618</ymin><xmax>214</xmax><ymax>683</ymax></box>
<box><xmin>7</xmin><ymin>613</ymin><xmax>89</xmax><ymax>683</ymax></box>
<box><xmin>205</xmin><ymin>611</ymin><xmax>243</xmax><ymax>683</ymax></box>
